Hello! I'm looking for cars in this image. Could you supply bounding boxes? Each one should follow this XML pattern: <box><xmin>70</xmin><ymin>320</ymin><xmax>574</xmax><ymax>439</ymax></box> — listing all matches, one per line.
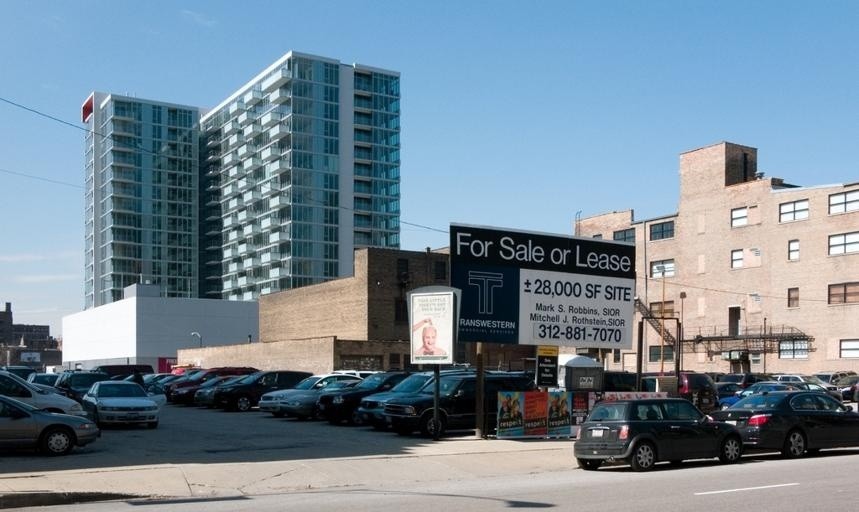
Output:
<box><xmin>601</xmin><ymin>369</ymin><xmax>859</xmax><ymax>411</ymax></box>
<box><xmin>1</xmin><ymin>395</ymin><xmax>101</xmax><ymax>457</ymax></box>
<box><xmin>707</xmin><ymin>391</ymin><xmax>859</xmax><ymax>459</ymax></box>
<box><xmin>573</xmin><ymin>396</ymin><xmax>743</xmax><ymax>471</ymax></box>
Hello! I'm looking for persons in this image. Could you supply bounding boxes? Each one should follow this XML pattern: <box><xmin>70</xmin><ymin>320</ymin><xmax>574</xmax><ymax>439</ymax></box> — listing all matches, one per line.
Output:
<box><xmin>498</xmin><ymin>394</ymin><xmax>512</xmax><ymax>419</ymax></box>
<box><xmin>558</xmin><ymin>400</ymin><xmax>570</xmax><ymax>425</ymax></box>
<box><xmin>510</xmin><ymin>399</ymin><xmax>522</xmax><ymax>426</ymax></box>
<box><xmin>411</xmin><ymin>319</ymin><xmax>433</xmax><ymax>332</ymax></box>
<box><xmin>413</xmin><ymin>327</ymin><xmax>448</xmax><ymax>356</ymax></box>
<box><xmin>549</xmin><ymin>395</ymin><xmax>562</xmax><ymax>420</ymax></box>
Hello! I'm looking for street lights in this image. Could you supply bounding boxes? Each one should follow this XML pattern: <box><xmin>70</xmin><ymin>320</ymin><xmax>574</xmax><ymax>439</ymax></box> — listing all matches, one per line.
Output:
<box><xmin>656</xmin><ymin>266</ymin><xmax>667</xmax><ymax>376</ymax></box>
